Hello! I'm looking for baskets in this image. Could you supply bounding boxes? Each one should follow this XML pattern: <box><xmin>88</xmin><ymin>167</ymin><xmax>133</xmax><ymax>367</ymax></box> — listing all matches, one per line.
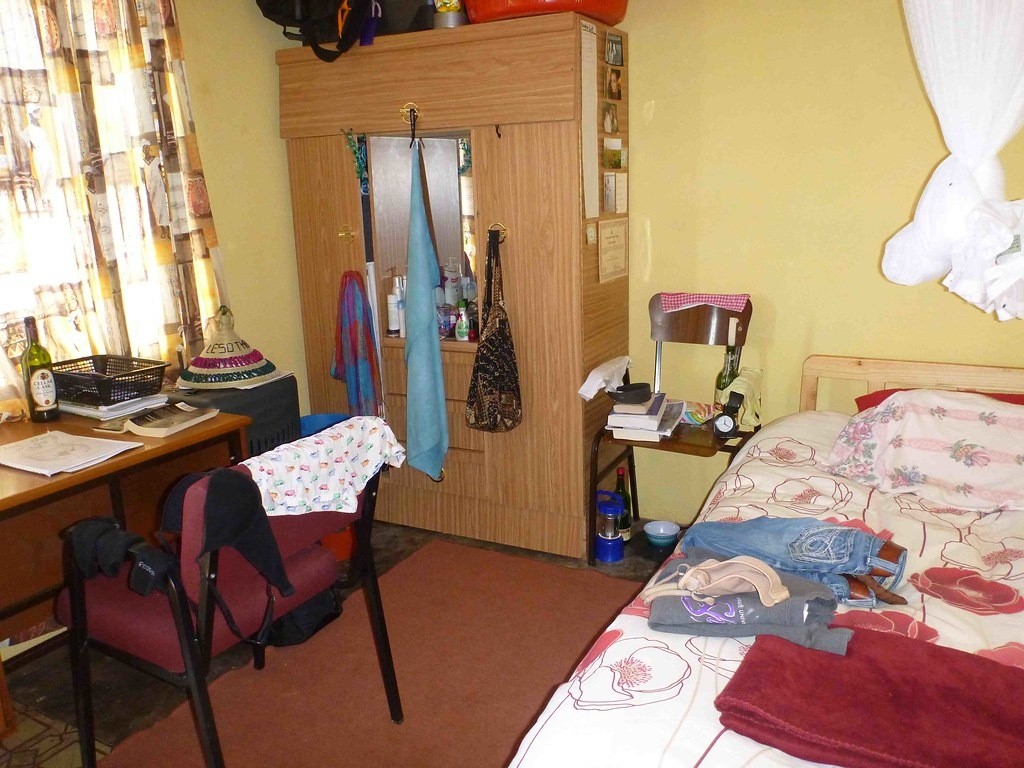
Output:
<box><xmin>31</xmin><ymin>354</ymin><xmax>171</xmax><ymax>406</ymax></box>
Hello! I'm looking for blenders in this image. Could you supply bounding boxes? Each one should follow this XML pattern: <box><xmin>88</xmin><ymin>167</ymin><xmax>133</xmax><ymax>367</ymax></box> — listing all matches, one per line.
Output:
<box><xmin>594</xmin><ymin>490</ymin><xmax>624</xmax><ymax>562</ymax></box>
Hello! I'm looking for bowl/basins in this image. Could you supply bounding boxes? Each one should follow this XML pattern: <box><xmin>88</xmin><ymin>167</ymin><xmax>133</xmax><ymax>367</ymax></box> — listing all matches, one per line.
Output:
<box><xmin>643</xmin><ymin>521</ymin><xmax>680</xmax><ymax>547</ymax></box>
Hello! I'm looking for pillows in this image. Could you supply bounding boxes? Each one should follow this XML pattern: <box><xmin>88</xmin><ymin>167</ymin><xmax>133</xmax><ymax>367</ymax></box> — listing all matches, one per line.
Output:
<box><xmin>811</xmin><ymin>388</ymin><xmax>1024</xmax><ymax>512</ymax></box>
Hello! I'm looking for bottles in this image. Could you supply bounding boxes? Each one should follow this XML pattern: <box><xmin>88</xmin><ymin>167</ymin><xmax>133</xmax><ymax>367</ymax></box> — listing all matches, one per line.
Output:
<box><xmin>611</xmin><ymin>467</ymin><xmax>631</xmax><ymax>545</ymax></box>
<box><xmin>713</xmin><ymin>346</ymin><xmax>740</xmax><ymax>432</ymax></box>
<box><xmin>21</xmin><ymin>316</ymin><xmax>59</xmax><ymax>422</ymax></box>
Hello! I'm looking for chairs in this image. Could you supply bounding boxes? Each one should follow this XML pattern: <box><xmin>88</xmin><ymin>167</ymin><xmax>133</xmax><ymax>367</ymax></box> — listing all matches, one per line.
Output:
<box><xmin>588</xmin><ymin>292</ymin><xmax>764</xmax><ymax>567</ymax></box>
<box><xmin>60</xmin><ymin>413</ymin><xmax>404</xmax><ymax>768</ymax></box>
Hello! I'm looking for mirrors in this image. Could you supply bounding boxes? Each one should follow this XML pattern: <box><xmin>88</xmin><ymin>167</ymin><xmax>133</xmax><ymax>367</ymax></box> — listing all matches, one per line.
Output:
<box><xmin>456</xmin><ymin>137</ymin><xmax>479</xmax><ymax>316</ymax></box>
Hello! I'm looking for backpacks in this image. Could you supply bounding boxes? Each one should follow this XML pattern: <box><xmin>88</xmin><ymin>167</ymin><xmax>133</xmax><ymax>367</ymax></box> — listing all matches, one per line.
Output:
<box><xmin>464</xmin><ymin>230</ymin><xmax>523</xmax><ymax>433</ymax></box>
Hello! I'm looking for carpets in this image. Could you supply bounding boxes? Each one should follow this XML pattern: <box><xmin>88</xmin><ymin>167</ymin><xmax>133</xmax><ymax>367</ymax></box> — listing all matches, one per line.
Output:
<box><xmin>93</xmin><ymin>536</ymin><xmax>643</xmax><ymax>768</ymax></box>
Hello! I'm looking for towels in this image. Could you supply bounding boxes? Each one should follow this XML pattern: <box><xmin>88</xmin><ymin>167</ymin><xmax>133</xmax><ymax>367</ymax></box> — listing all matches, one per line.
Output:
<box><xmin>406</xmin><ymin>134</ymin><xmax>451</xmax><ymax>479</ymax></box>
<box><xmin>714</xmin><ymin>625</ymin><xmax>1024</xmax><ymax>768</ymax></box>
<box><xmin>828</xmin><ymin>388</ymin><xmax>1023</xmax><ymax>512</ymax></box>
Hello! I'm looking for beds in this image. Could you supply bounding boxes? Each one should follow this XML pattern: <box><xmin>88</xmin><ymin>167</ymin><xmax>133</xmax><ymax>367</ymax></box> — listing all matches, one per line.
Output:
<box><xmin>505</xmin><ymin>355</ymin><xmax>1024</xmax><ymax>768</ymax></box>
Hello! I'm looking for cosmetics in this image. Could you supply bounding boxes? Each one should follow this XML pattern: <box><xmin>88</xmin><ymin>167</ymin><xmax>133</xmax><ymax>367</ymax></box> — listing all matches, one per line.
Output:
<box><xmin>385</xmin><ymin>255</ymin><xmax>476</xmax><ymax>341</ymax></box>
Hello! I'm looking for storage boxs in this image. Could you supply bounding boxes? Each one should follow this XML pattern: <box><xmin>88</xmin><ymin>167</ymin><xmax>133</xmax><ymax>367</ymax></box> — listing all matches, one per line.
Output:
<box><xmin>51</xmin><ymin>355</ymin><xmax>172</xmax><ymax>406</ymax></box>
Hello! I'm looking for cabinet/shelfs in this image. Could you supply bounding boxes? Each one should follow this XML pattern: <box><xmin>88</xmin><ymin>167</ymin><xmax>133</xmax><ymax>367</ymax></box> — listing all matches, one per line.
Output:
<box><xmin>272</xmin><ymin>9</ymin><xmax>630</xmax><ymax>565</ymax></box>
<box><xmin>156</xmin><ymin>373</ymin><xmax>303</xmax><ymax>458</ymax></box>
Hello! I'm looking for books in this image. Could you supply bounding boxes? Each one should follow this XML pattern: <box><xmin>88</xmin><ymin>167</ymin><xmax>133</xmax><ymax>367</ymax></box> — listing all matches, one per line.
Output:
<box><xmin>604</xmin><ymin>390</ymin><xmax>685</xmax><ymax>442</ymax></box>
<box><xmin>92</xmin><ymin>400</ymin><xmax>220</xmax><ymax>438</ymax></box>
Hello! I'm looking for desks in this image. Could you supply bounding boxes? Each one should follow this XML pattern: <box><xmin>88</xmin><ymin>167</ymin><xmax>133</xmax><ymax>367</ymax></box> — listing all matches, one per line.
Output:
<box><xmin>0</xmin><ymin>397</ymin><xmax>251</xmax><ymax>742</ymax></box>
